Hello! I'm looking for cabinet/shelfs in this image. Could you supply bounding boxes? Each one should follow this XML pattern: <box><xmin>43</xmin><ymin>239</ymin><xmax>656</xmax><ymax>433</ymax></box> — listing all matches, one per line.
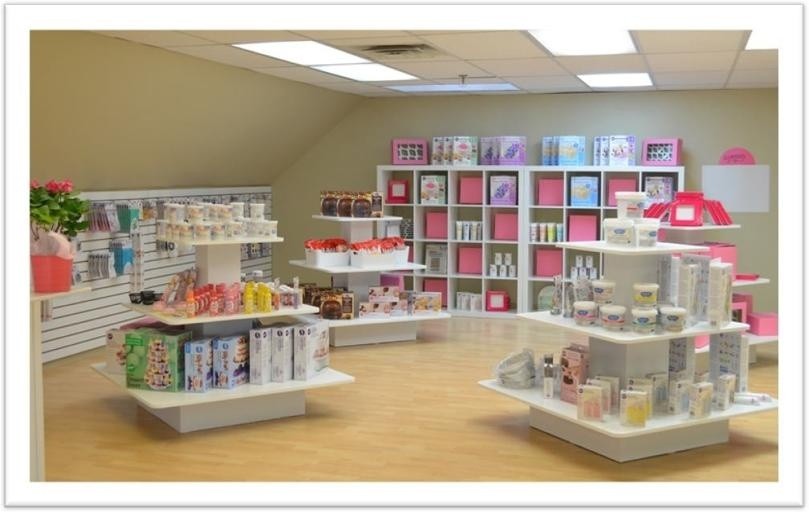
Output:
<box><xmin>477</xmin><ymin>222</ymin><xmax>779</xmax><ymax>464</ymax></box>
<box><xmin>374</xmin><ymin>164</ymin><xmax>686</xmax><ymax>318</ymax></box>
<box><xmin>90</xmin><ymin>230</ymin><xmax>358</xmax><ymax>434</ymax></box>
<box><xmin>286</xmin><ymin>214</ymin><xmax>450</xmax><ymax>348</ymax></box>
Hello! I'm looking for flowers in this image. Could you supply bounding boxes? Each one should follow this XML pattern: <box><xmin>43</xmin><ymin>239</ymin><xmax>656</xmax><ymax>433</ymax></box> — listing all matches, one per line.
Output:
<box><xmin>30</xmin><ymin>177</ymin><xmax>91</xmax><ymax>241</ymax></box>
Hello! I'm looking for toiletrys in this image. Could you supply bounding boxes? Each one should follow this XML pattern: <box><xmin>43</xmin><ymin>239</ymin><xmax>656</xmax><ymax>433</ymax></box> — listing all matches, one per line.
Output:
<box><xmin>531</xmin><ymin>223</ymin><xmax>563</xmax><ymax>243</ymax></box>
<box><xmin>543</xmin><ymin>192</ymin><xmax>768</xmax><ymax>425</ymax></box>
<box><xmin>630</xmin><ymin>254</ymin><xmax>735</xmax><ymax>335</ymax></box>
<box><xmin>613</xmin><ymin>192</ymin><xmax>648</xmax><ymax>219</ymax></box>
<box><xmin>603</xmin><ymin>217</ymin><xmax>662</xmax><ymax>249</ymax></box>
<box><xmin>455</xmin><ymin>220</ymin><xmax>481</xmax><ymax>240</ymax></box>
<box><xmin>549</xmin><ymin>248</ymin><xmax>627</xmax><ymax>329</ymax></box>
<box><xmin>618</xmin><ymin>335</ymin><xmax>768</xmax><ymax>425</ymax></box>
<box><xmin>544</xmin><ymin>343</ymin><xmax>620</xmax><ymax>425</ymax></box>
<box><xmin>457</xmin><ymin>292</ymin><xmax>483</xmax><ymax>311</ymax></box>
